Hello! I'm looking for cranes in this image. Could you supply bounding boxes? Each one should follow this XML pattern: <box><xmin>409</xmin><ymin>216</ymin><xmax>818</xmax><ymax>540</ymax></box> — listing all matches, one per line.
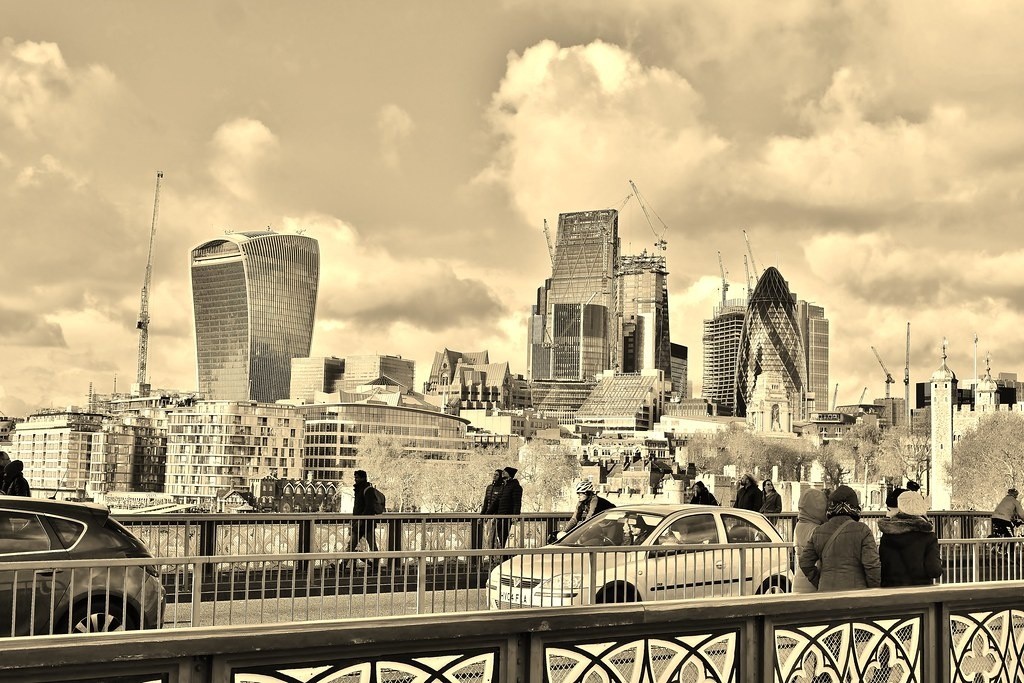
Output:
<box><xmin>628</xmin><ymin>179</ymin><xmax>669</xmax><ymax>256</ymax></box>
<box><xmin>716</xmin><ymin>249</ymin><xmax>730</xmax><ymax>308</ymax></box>
<box><xmin>871</xmin><ymin>345</ymin><xmax>896</xmax><ymax>398</ymax></box>
<box><xmin>742</xmin><ymin>229</ymin><xmax>766</xmax><ymax>283</ymax></box>
<box><xmin>136</xmin><ymin>169</ymin><xmax>167</xmax><ymax>385</ymax></box>
<box><xmin>831</xmin><ymin>383</ymin><xmax>839</xmax><ymax>411</ymax></box>
<box><xmin>858</xmin><ymin>387</ymin><xmax>868</xmax><ymax>404</ymax></box>
<box><xmin>742</xmin><ymin>253</ymin><xmax>754</xmax><ymax>299</ymax></box>
<box><xmin>542</xmin><ymin>218</ymin><xmax>555</xmax><ymax>267</ymax></box>
<box><xmin>902</xmin><ymin>322</ymin><xmax>911</xmax><ymax>428</ymax></box>
<box><xmin>595</xmin><ymin>194</ymin><xmax>634</xmax><ymax>307</ymax></box>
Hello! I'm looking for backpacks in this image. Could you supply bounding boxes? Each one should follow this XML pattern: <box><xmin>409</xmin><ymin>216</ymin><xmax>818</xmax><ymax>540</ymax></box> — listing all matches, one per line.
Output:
<box><xmin>363</xmin><ymin>485</ymin><xmax>385</xmax><ymax>514</ymax></box>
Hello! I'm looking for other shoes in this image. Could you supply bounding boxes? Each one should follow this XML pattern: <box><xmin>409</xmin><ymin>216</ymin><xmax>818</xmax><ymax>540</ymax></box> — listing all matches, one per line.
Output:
<box><xmin>330</xmin><ymin>562</ymin><xmax>345</xmax><ymax>573</ymax></box>
<box><xmin>372</xmin><ymin>559</ymin><xmax>380</xmax><ymax>574</ymax></box>
<box><xmin>1007</xmin><ymin>557</ymin><xmax>1015</xmax><ymax>563</ymax></box>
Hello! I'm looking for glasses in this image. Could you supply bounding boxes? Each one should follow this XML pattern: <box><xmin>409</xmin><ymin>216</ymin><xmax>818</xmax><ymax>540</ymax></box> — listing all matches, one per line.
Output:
<box><xmin>765</xmin><ymin>483</ymin><xmax>773</xmax><ymax>486</ymax></box>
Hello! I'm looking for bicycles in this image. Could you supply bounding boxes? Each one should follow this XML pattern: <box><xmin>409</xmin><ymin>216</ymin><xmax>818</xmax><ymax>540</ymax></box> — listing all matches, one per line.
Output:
<box><xmin>982</xmin><ymin>519</ymin><xmax>1024</xmax><ymax>570</ymax></box>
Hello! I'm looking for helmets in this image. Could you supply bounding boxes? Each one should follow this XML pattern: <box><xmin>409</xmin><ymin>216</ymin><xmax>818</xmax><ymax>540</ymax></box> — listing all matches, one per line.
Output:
<box><xmin>576</xmin><ymin>480</ymin><xmax>593</xmax><ymax>493</ymax></box>
<box><xmin>1008</xmin><ymin>488</ymin><xmax>1018</xmax><ymax>496</ymax></box>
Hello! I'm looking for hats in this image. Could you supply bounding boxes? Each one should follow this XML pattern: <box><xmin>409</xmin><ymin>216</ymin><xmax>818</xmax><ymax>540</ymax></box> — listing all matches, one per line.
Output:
<box><xmin>831</xmin><ymin>485</ymin><xmax>859</xmax><ymax>507</ymax></box>
<box><xmin>897</xmin><ymin>491</ymin><xmax>927</xmax><ymax>515</ymax></box>
<box><xmin>504</xmin><ymin>467</ymin><xmax>518</xmax><ymax>479</ymax></box>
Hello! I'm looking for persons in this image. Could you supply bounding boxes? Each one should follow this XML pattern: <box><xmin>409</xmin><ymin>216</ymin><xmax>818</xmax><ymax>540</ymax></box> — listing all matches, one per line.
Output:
<box><xmin>991</xmin><ymin>488</ymin><xmax>1024</xmax><ymax>563</ymax></box>
<box><xmin>689</xmin><ymin>481</ymin><xmax>717</xmax><ymax>505</ymax></box>
<box><xmin>0</xmin><ymin>451</ymin><xmax>31</xmax><ymax>497</ymax></box>
<box><xmin>791</xmin><ymin>480</ymin><xmax>943</xmax><ymax>683</ymax></box>
<box><xmin>478</xmin><ymin>467</ymin><xmax>522</xmax><ymax>564</ymax></box>
<box><xmin>733</xmin><ymin>473</ymin><xmax>763</xmax><ymax>512</ymax></box>
<box><xmin>556</xmin><ymin>479</ymin><xmax>623</xmax><ymax>545</ymax></box>
<box><xmin>759</xmin><ymin>479</ymin><xmax>782</xmax><ymax>526</ymax></box>
<box><xmin>628</xmin><ymin>515</ymin><xmax>659</xmax><ymax>556</ymax></box>
<box><xmin>330</xmin><ymin>470</ymin><xmax>379</xmax><ymax>574</ymax></box>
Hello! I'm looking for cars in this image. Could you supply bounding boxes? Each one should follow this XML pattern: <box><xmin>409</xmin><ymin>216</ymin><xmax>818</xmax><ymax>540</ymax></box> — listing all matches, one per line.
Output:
<box><xmin>0</xmin><ymin>490</ymin><xmax>166</xmax><ymax>639</ymax></box>
<box><xmin>485</xmin><ymin>503</ymin><xmax>794</xmax><ymax>610</ymax></box>
